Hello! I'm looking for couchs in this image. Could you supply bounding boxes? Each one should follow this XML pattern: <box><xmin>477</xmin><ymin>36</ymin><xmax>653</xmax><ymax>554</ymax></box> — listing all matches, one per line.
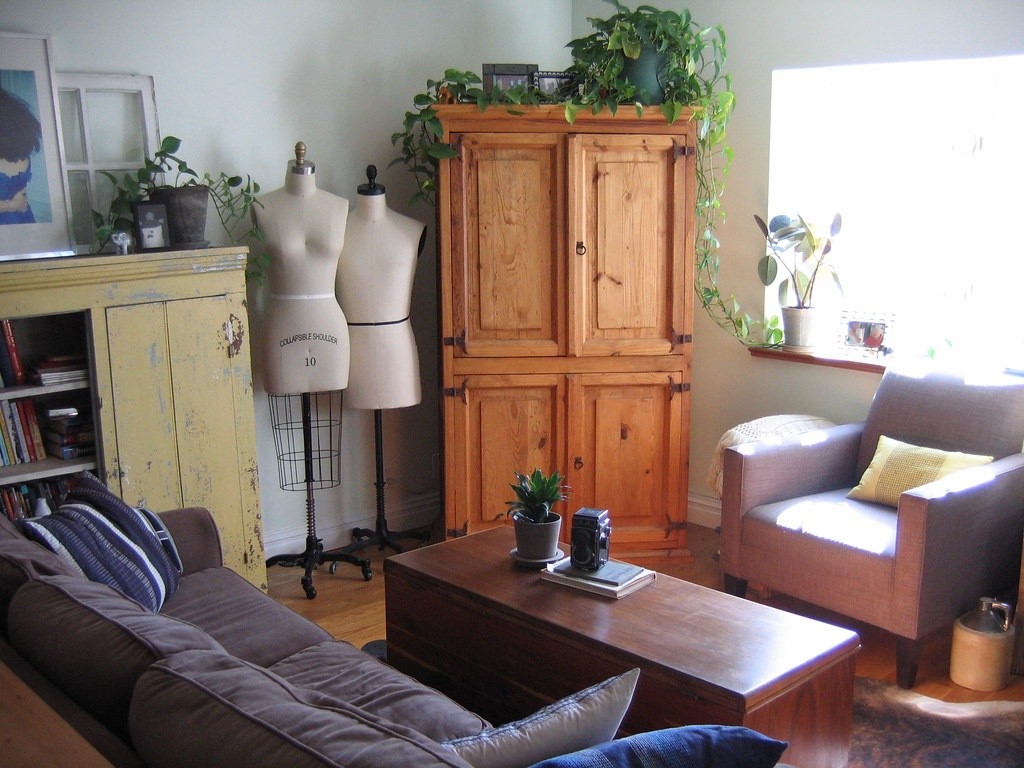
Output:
<box><xmin>0</xmin><ymin>497</ymin><xmax>800</xmax><ymax>768</ymax></box>
<box><xmin>721</xmin><ymin>367</ymin><xmax>1024</xmax><ymax>689</ymax></box>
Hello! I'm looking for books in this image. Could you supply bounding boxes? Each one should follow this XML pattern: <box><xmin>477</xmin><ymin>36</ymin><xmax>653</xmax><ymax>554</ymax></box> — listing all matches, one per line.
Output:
<box><xmin>0</xmin><ymin>319</ymin><xmax>96</xmax><ymax>521</ymax></box>
<box><xmin>540</xmin><ymin>554</ymin><xmax>657</xmax><ymax>600</ymax></box>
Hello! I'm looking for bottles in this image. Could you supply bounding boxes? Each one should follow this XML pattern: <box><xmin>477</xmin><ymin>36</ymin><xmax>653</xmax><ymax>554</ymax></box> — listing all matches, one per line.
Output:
<box><xmin>35</xmin><ymin>497</ymin><xmax>52</xmax><ymax>516</ymax></box>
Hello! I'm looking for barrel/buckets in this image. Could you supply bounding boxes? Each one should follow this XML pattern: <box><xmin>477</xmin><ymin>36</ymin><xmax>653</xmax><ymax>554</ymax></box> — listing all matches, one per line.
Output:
<box><xmin>949</xmin><ymin>596</ymin><xmax>1016</xmax><ymax>692</ymax></box>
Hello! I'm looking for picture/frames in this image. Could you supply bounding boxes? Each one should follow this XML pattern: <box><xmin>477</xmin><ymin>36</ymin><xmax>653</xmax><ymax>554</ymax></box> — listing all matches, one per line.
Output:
<box><xmin>0</xmin><ymin>32</ymin><xmax>79</xmax><ymax>261</ymax></box>
<box><xmin>533</xmin><ymin>72</ymin><xmax>575</xmax><ymax>103</ymax></box>
<box><xmin>481</xmin><ymin>64</ymin><xmax>539</xmax><ymax>102</ymax></box>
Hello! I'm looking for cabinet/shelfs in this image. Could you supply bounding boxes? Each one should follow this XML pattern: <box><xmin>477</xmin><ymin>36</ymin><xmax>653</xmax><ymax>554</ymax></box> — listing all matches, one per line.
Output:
<box><xmin>424</xmin><ymin>98</ymin><xmax>697</xmax><ymax>554</ymax></box>
<box><xmin>0</xmin><ymin>245</ymin><xmax>270</xmax><ymax>599</ymax></box>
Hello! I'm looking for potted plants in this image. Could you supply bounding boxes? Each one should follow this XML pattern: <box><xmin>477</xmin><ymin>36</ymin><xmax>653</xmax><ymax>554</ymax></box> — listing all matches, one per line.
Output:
<box><xmin>504</xmin><ymin>469</ymin><xmax>572</xmax><ymax>572</ymax></box>
<box><xmin>378</xmin><ymin>1</ymin><xmax>786</xmax><ymax>349</ymax></box>
<box><xmin>87</xmin><ymin>135</ymin><xmax>274</xmax><ymax>291</ymax></box>
<box><xmin>754</xmin><ymin>213</ymin><xmax>848</xmax><ymax>348</ymax></box>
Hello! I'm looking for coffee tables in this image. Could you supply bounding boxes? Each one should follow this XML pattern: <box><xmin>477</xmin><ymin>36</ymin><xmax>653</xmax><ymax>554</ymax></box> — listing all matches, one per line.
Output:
<box><xmin>383</xmin><ymin>525</ymin><xmax>860</xmax><ymax>768</ymax></box>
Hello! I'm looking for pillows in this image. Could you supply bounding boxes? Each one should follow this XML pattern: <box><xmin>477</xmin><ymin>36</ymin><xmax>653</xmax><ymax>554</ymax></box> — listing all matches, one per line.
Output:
<box><xmin>847</xmin><ymin>436</ymin><xmax>994</xmax><ymax>510</ymax></box>
<box><xmin>15</xmin><ymin>472</ymin><xmax>183</xmax><ymax>615</ymax></box>
<box><xmin>445</xmin><ymin>663</ymin><xmax>638</xmax><ymax>768</ymax></box>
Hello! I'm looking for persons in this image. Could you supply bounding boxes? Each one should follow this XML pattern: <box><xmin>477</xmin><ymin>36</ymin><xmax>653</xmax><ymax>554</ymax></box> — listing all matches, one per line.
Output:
<box><xmin>250</xmin><ymin>160</ymin><xmax>349</xmax><ymax>395</ymax></box>
<box><xmin>334</xmin><ymin>184</ymin><xmax>426</xmax><ymax>409</ymax></box>
<box><xmin>847</xmin><ymin>321</ymin><xmax>884</xmax><ymax>349</ymax></box>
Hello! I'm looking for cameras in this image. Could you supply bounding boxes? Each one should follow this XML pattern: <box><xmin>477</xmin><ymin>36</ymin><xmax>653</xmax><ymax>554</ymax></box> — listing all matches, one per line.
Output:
<box><xmin>570</xmin><ymin>507</ymin><xmax>612</xmax><ymax>572</ymax></box>
